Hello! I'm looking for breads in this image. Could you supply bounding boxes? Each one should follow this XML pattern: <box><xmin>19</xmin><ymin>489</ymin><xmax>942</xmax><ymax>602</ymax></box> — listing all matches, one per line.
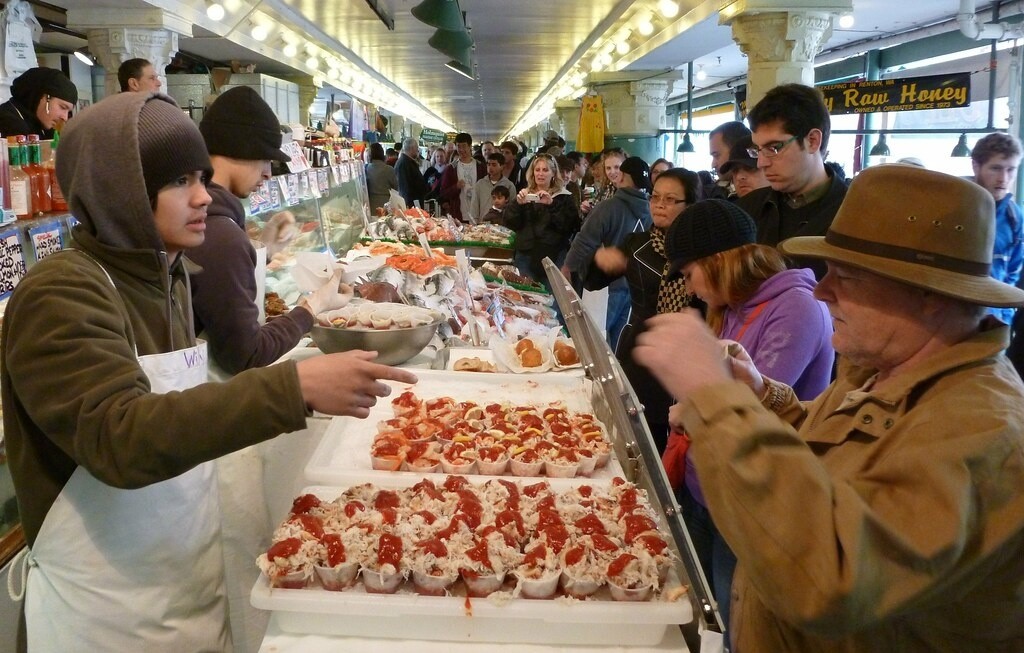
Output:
<box><xmin>454</xmin><ymin>358</ymin><xmax>498</xmax><ymax>372</ymax></box>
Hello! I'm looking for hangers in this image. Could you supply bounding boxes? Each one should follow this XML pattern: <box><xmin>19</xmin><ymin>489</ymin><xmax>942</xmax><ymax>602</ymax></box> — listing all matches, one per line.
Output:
<box><xmin>586</xmin><ymin>85</ymin><xmax>598</xmax><ymax>97</ymax></box>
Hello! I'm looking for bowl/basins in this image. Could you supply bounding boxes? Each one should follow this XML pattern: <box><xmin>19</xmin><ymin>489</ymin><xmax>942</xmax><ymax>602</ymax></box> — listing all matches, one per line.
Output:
<box><xmin>267</xmin><ymin>396</ymin><xmax>653</xmax><ymax>602</ymax></box>
<box><xmin>312</xmin><ymin>307</ymin><xmax>446</xmax><ymax>366</ymax></box>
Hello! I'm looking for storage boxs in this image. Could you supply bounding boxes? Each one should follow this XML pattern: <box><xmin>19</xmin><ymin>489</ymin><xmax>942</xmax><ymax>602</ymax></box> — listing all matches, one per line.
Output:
<box><xmin>305</xmin><ymin>377</ymin><xmax>629</xmax><ymax>486</ymax></box>
<box><xmin>251</xmin><ymin>485</ymin><xmax>693</xmax><ymax>646</ymax></box>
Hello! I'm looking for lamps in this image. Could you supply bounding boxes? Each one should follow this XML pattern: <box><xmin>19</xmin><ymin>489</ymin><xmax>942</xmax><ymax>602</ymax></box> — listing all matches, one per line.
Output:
<box><xmin>868</xmin><ymin>134</ymin><xmax>890</xmax><ymax>156</ymax></box>
<box><xmin>951</xmin><ymin>133</ymin><xmax>972</xmax><ymax>157</ymax></box>
<box><xmin>445</xmin><ymin>59</ymin><xmax>475</xmax><ymax>81</ymax></box>
<box><xmin>697</xmin><ymin>64</ymin><xmax>706</xmax><ymax>81</ymax></box>
<box><xmin>677</xmin><ymin>133</ymin><xmax>694</xmax><ymax>152</ymax></box>
<box><xmin>411</xmin><ymin>0</ymin><xmax>463</xmax><ymax>31</ymax></box>
<box><xmin>74</xmin><ymin>49</ymin><xmax>94</xmax><ymax>65</ymax></box>
<box><xmin>427</xmin><ymin>28</ymin><xmax>474</xmax><ymax>51</ymax></box>
<box><xmin>443</xmin><ymin>48</ymin><xmax>470</xmax><ymax>68</ymax></box>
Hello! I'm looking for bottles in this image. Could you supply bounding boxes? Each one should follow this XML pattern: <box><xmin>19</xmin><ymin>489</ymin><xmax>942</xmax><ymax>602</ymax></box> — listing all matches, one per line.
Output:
<box><xmin>16</xmin><ymin>136</ymin><xmax>40</xmax><ymax>214</ymax></box>
<box><xmin>305</xmin><ymin>137</ymin><xmax>355</xmax><ymax>164</ymax></box>
<box><xmin>28</xmin><ymin>135</ymin><xmax>52</xmax><ymax>210</ymax></box>
<box><xmin>7</xmin><ymin>136</ymin><xmax>32</xmax><ymax>219</ymax></box>
<box><xmin>0</xmin><ymin>133</ymin><xmax>12</xmax><ymax>209</ymax></box>
<box><xmin>41</xmin><ymin>129</ymin><xmax>67</xmax><ymax>210</ymax></box>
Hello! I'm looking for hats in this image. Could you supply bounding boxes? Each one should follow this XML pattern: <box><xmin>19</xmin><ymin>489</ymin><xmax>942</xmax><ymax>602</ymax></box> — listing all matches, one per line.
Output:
<box><xmin>370</xmin><ymin>143</ymin><xmax>384</xmax><ymax>160</ymax></box>
<box><xmin>776</xmin><ymin>163</ymin><xmax>1024</xmax><ymax>308</ymax></box>
<box><xmin>619</xmin><ymin>156</ymin><xmax>653</xmax><ymax>188</ymax></box>
<box><xmin>665</xmin><ymin>199</ymin><xmax>758</xmax><ymax>287</ymax></box>
<box><xmin>199</xmin><ymin>87</ymin><xmax>292</xmax><ymax>162</ymax></box>
<box><xmin>557</xmin><ymin>155</ymin><xmax>576</xmax><ymax>171</ymax></box>
<box><xmin>719</xmin><ymin>136</ymin><xmax>757</xmax><ymax>175</ymax></box>
<box><xmin>543</xmin><ymin>130</ymin><xmax>559</xmax><ymax>142</ymax></box>
<box><xmin>138</xmin><ymin>98</ymin><xmax>214</xmax><ymax>194</ymax></box>
<box><xmin>44</xmin><ymin>74</ymin><xmax>77</xmax><ymax>105</ymax></box>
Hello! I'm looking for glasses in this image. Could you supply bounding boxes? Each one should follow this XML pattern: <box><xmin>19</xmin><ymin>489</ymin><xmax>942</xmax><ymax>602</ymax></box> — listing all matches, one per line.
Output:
<box><xmin>534</xmin><ymin>154</ymin><xmax>556</xmax><ymax>168</ymax></box>
<box><xmin>604</xmin><ymin>147</ymin><xmax>623</xmax><ymax>155</ymax></box>
<box><xmin>648</xmin><ymin>194</ymin><xmax>687</xmax><ymax>206</ymax></box>
<box><xmin>746</xmin><ymin>128</ymin><xmax>823</xmax><ymax>158</ymax></box>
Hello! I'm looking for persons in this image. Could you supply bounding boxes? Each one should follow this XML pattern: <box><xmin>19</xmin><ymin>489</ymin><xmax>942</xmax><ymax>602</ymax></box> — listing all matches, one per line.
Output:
<box><xmin>387</xmin><ymin>132</ymin><xmax>631</xmax><ymax>288</ymax></box>
<box><xmin>118</xmin><ymin>57</ymin><xmax>162</xmax><ymax>92</ymax></box>
<box><xmin>562</xmin><ymin>82</ymin><xmax>1024</xmax><ymax>653</ymax></box>
<box><xmin>0</xmin><ymin>67</ymin><xmax>78</xmax><ymax>141</ymax></box>
<box><xmin>184</xmin><ymin>84</ymin><xmax>354</xmax><ymax>653</ymax></box>
<box><xmin>0</xmin><ymin>89</ymin><xmax>419</xmax><ymax>653</ymax></box>
<box><xmin>365</xmin><ymin>142</ymin><xmax>399</xmax><ymax>216</ymax></box>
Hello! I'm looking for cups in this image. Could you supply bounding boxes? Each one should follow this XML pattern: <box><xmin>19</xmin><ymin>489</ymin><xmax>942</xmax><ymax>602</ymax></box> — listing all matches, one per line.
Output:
<box><xmin>584</xmin><ymin>187</ymin><xmax>594</xmax><ymax>202</ymax></box>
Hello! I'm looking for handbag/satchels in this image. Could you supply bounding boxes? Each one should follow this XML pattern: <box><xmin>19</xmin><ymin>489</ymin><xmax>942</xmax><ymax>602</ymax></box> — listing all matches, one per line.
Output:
<box><xmin>661</xmin><ymin>428</ymin><xmax>691</xmax><ymax>490</ymax></box>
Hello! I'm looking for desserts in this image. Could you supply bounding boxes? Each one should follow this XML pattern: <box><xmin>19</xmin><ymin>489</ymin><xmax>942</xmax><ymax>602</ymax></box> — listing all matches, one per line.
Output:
<box><xmin>255</xmin><ymin>391</ymin><xmax>669</xmax><ymax>602</ymax></box>
<box><xmin>515</xmin><ymin>338</ymin><xmax>579</xmax><ymax>367</ymax></box>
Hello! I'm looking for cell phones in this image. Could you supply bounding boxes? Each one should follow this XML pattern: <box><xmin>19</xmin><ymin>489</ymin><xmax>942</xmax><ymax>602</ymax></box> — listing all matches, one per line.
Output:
<box><xmin>524</xmin><ymin>194</ymin><xmax>540</xmax><ymax>201</ymax></box>
